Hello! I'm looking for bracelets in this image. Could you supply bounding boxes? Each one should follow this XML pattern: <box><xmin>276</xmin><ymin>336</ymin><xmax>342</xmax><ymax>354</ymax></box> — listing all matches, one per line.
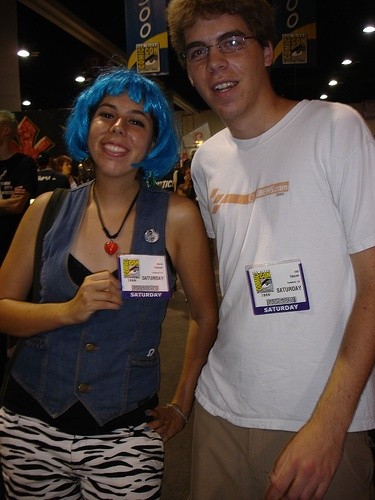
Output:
<box><xmin>169</xmin><ymin>403</ymin><xmax>190</xmax><ymax>427</ymax></box>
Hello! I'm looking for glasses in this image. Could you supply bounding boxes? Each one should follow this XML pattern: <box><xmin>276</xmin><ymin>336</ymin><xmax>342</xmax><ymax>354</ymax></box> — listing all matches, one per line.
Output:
<box><xmin>181</xmin><ymin>34</ymin><xmax>262</xmax><ymax>63</ymax></box>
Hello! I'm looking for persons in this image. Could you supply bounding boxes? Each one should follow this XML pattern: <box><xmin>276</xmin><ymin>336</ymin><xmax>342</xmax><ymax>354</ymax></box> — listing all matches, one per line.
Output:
<box><xmin>35</xmin><ymin>150</ymin><xmax>71</xmax><ymax>197</ymax></box>
<box><xmin>1</xmin><ymin>68</ymin><xmax>219</xmax><ymax>498</ymax></box>
<box><xmin>166</xmin><ymin>1</ymin><xmax>375</xmax><ymax>500</ymax></box>
<box><xmin>175</xmin><ymin>159</ymin><xmax>199</xmax><ymax>209</ymax></box>
<box><xmin>1</xmin><ymin>108</ymin><xmax>39</xmax><ymax>266</ymax></box>
<box><xmin>53</xmin><ymin>156</ymin><xmax>78</xmax><ymax>190</ymax></box>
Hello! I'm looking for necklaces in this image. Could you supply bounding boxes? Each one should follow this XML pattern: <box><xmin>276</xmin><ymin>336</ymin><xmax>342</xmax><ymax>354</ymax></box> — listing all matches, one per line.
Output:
<box><xmin>91</xmin><ymin>180</ymin><xmax>143</xmax><ymax>256</ymax></box>
